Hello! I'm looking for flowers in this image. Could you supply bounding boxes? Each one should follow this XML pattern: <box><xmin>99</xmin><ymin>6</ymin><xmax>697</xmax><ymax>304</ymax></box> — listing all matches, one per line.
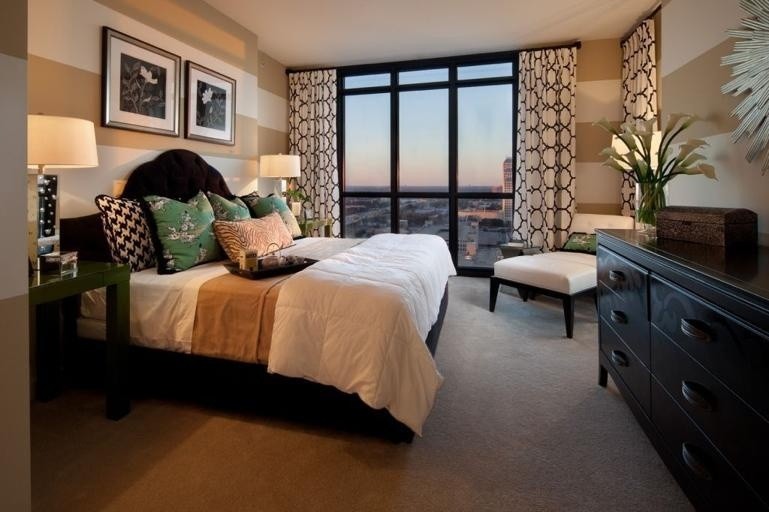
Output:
<box><xmin>593</xmin><ymin>113</ymin><xmax>719</xmax><ymax>225</ymax></box>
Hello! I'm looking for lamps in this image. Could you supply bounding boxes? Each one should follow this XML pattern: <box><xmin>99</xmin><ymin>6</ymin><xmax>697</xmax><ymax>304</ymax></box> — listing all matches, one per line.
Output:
<box><xmin>258</xmin><ymin>152</ymin><xmax>301</xmax><ymax>204</ymax></box>
<box><xmin>28</xmin><ymin>109</ymin><xmax>100</xmax><ymax>280</ymax></box>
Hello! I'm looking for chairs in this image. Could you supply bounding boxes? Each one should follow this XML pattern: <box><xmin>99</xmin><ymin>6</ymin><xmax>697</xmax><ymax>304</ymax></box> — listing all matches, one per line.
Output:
<box><xmin>489</xmin><ymin>214</ymin><xmax>649</xmax><ymax>339</ymax></box>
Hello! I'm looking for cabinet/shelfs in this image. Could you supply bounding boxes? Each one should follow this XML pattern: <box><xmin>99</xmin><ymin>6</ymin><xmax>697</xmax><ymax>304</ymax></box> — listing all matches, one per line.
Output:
<box><xmin>593</xmin><ymin>227</ymin><xmax>768</xmax><ymax>509</ymax></box>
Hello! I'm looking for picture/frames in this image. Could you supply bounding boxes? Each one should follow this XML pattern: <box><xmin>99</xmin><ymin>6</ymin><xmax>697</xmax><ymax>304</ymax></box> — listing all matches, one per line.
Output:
<box><xmin>184</xmin><ymin>62</ymin><xmax>237</xmax><ymax>145</ymax></box>
<box><xmin>99</xmin><ymin>22</ymin><xmax>184</xmax><ymax>139</ymax></box>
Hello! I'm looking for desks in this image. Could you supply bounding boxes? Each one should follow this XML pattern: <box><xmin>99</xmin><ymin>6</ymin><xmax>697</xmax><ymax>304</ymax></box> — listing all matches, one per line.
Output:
<box><xmin>29</xmin><ymin>261</ymin><xmax>135</xmax><ymax>422</ymax></box>
<box><xmin>298</xmin><ymin>219</ymin><xmax>334</xmax><ymax>239</ymax></box>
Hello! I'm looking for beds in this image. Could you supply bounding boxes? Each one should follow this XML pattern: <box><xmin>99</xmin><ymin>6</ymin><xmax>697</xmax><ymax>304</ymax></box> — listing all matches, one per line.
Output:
<box><xmin>58</xmin><ymin>148</ymin><xmax>458</xmax><ymax>448</ymax></box>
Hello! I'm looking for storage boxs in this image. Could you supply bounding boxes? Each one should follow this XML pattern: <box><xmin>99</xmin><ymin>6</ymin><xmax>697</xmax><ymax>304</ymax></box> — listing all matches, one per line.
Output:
<box><xmin>656</xmin><ymin>205</ymin><xmax>759</xmax><ymax>250</ymax></box>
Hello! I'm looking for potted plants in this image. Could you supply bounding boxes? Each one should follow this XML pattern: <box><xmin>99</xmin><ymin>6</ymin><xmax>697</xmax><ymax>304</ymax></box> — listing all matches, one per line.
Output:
<box><xmin>284</xmin><ymin>189</ymin><xmax>309</xmax><ymax>216</ymax></box>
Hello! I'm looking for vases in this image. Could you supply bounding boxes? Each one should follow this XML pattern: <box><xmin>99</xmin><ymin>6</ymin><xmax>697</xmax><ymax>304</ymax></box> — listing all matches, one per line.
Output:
<box><xmin>635</xmin><ymin>183</ymin><xmax>660</xmax><ymax>236</ymax></box>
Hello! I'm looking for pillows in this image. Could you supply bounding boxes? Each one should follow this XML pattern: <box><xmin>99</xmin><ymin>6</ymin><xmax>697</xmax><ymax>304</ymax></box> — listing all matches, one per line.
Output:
<box><xmin>96</xmin><ymin>186</ymin><xmax>305</xmax><ymax>275</ymax></box>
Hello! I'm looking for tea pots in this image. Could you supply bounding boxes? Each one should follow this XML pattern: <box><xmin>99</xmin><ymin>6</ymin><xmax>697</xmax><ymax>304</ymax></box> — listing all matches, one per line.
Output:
<box><xmin>262</xmin><ymin>241</ymin><xmax>282</xmax><ymax>268</ymax></box>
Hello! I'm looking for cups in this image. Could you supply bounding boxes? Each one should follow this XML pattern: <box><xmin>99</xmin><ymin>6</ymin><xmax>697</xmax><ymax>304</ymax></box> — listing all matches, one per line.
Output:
<box><xmin>279</xmin><ymin>255</ymin><xmax>305</xmax><ymax>267</ymax></box>
